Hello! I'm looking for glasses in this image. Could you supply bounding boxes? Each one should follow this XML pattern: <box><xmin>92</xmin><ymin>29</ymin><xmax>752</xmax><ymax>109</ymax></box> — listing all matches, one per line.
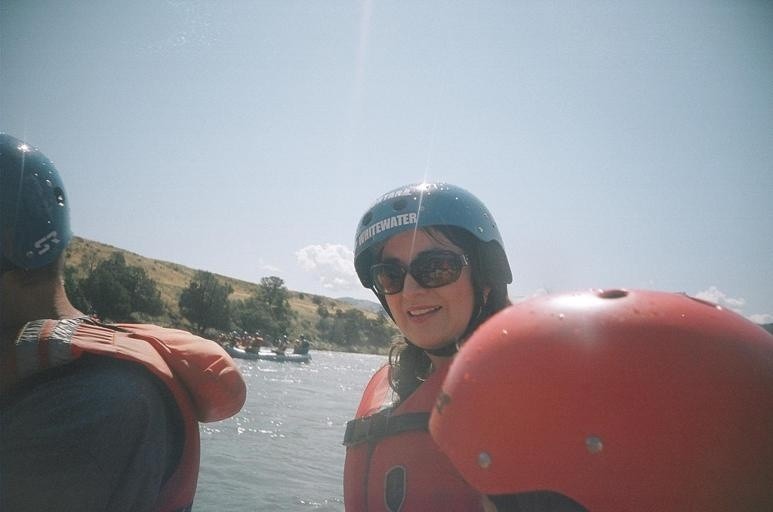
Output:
<box><xmin>368</xmin><ymin>248</ymin><xmax>473</xmax><ymax>295</ymax></box>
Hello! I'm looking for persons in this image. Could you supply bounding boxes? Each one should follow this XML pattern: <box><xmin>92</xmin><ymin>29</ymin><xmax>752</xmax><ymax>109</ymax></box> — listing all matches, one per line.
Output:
<box><xmin>217</xmin><ymin>330</ymin><xmax>310</xmax><ymax>356</ymax></box>
<box><xmin>340</xmin><ymin>181</ymin><xmax>513</xmax><ymax>512</ymax></box>
<box><xmin>0</xmin><ymin>133</ymin><xmax>247</xmax><ymax>512</ymax></box>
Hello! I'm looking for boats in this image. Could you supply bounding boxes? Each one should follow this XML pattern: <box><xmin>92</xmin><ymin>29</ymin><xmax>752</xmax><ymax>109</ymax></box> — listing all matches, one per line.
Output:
<box><xmin>223</xmin><ymin>340</ymin><xmax>311</xmax><ymax>363</ymax></box>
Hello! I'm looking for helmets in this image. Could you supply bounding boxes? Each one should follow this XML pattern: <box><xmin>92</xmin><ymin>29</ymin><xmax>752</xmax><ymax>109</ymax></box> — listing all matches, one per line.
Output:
<box><xmin>0</xmin><ymin>133</ymin><xmax>69</xmax><ymax>272</ymax></box>
<box><xmin>353</xmin><ymin>180</ymin><xmax>514</xmax><ymax>288</ymax></box>
